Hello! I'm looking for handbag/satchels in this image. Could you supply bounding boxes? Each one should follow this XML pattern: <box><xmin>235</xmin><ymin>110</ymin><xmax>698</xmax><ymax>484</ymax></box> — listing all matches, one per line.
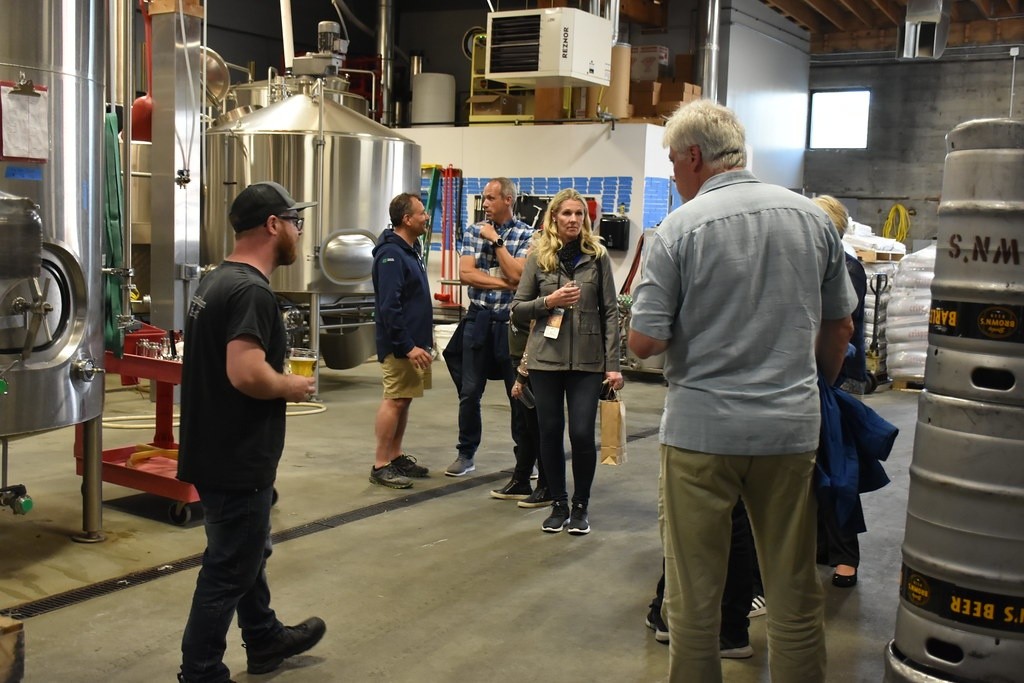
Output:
<box><xmin>599</xmin><ymin>386</ymin><xmax>629</xmax><ymax>466</ymax></box>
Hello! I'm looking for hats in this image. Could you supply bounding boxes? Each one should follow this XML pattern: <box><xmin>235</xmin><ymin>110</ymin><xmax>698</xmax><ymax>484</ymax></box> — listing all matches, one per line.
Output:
<box><xmin>229</xmin><ymin>181</ymin><xmax>319</xmax><ymax>233</ymax></box>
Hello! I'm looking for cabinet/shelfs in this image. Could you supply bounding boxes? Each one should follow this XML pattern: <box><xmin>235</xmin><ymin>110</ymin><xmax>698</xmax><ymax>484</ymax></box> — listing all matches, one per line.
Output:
<box><xmin>466</xmin><ymin>34</ymin><xmax>571</xmax><ymax>126</ymax></box>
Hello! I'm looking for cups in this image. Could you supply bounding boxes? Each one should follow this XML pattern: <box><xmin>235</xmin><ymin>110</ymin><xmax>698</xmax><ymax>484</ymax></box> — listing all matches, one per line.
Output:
<box><xmin>289</xmin><ymin>356</ymin><xmax>318</xmax><ymax>377</ymax></box>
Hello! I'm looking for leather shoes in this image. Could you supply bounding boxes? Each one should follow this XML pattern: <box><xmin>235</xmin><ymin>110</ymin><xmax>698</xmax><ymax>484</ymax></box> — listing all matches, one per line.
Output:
<box><xmin>832</xmin><ymin>567</ymin><xmax>857</xmax><ymax>588</ymax></box>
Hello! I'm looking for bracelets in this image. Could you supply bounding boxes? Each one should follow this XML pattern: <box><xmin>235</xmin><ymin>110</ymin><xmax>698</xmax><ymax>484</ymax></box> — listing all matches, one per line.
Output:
<box><xmin>544</xmin><ymin>296</ymin><xmax>551</xmax><ymax>310</ymax></box>
<box><xmin>514</xmin><ymin>380</ymin><xmax>523</xmax><ymax>389</ymax></box>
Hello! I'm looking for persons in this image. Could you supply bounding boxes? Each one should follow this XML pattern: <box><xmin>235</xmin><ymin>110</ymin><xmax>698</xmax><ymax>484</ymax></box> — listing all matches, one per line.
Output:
<box><xmin>629</xmin><ymin>99</ymin><xmax>859</xmax><ymax>683</ymax></box>
<box><xmin>445</xmin><ymin>177</ymin><xmax>539</xmax><ymax>479</ymax></box>
<box><xmin>490</xmin><ymin>229</ymin><xmax>555</xmax><ymax>508</ymax></box>
<box><xmin>512</xmin><ymin>188</ymin><xmax>624</xmax><ymax>534</ymax></box>
<box><xmin>175</xmin><ymin>179</ymin><xmax>326</xmax><ymax>683</ymax></box>
<box><xmin>369</xmin><ymin>193</ymin><xmax>433</xmax><ymax>489</ymax></box>
<box><xmin>644</xmin><ymin>194</ymin><xmax>866</xmax><ymax>658</ymax></box>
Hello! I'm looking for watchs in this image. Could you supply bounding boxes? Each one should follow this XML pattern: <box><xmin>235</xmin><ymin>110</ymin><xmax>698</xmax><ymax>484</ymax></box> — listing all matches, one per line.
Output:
<box><xmin>492</xmin><ymin>238</ymin><xmax>504</xmax><ymax>249</ymax></box>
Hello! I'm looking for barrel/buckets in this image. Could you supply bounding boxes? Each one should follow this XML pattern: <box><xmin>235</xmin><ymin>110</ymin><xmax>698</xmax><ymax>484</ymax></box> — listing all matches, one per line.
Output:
<box><xmin>885</xmin><ymin>122</ymin><xmax>1024</xmax><ymax>683</ymax></box>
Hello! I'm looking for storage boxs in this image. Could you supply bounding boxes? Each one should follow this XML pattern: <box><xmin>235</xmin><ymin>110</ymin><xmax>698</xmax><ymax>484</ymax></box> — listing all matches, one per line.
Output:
<box><xmin>466</xmin><ymin>95</ymin><xmax>517</xmax><ymax>115</ymax></box>
<box><xmin>629</xmin><ymin>77</ymin><xmax>702</xmax><ymax>116</ymax></box>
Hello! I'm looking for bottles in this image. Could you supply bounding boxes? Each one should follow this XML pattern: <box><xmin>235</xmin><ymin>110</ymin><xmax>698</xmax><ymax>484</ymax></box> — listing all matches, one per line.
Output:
<box><xmin>136</xmin><ymin>337</ymin><xmax>184</xmax><ymax>363</ymax></box>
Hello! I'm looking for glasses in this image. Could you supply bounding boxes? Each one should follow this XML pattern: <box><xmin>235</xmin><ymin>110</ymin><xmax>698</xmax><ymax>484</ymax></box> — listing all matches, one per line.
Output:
<box><xmin>264</xmin><ymin>216</ymin><xmax>304</xmax><ymax>231</ymax></box>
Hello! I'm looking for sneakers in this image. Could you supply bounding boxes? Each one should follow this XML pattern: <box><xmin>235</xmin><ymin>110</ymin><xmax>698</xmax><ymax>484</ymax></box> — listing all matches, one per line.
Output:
<box><xmin>720</xmin><ymin>632</ymin><xmax>753</xmax><ymax>658</ymax></box>
<box><xmin>490</xmin><ymin>479</ymin><xmax>533</xmax><ymax>500</ymax></box>
<box><xmin>241</xmin><ymin>617</ymin><xmax>327</xmax><ymax>674</ymax></box>
<box><xmin>517</xmin><ymin>487</ymin><xmax>554</xmax><ymax>508</ymax></box>
<box><xmin>445</xmin><ymin>453</ymin><xmax>475</xmax><ymax>477</ymax></box>
<box><xmin>368</xmin><ymin>462</ymin><xmax>414</xmax><ymax>489</ymax></box>
<box><xmin>568</xmin><ymin>504</ymin><xmax>591</xmax><ymax>534</ymax></box>
<box><xmin>646</xmin><ymin>604</ymin><xmax>669</xmax><ymax>641</ymax></box>
<box><xmin>177</xmin><ymin>673</ymin><xmax>237</xmax><ymax>683</ymax></box>
<box><xmin>542</xmin><ymin>502</ymin><xmax>571</xmax><ymax>532</ymax></box>
<box><xmin>530</xmin><ymin>465</ymin><xmax>538</xmax><ymax>479</ymax></box>
<box><xmin>747</xmin><ymin>595</ymin><xmax>767</xmax><ymax>618</ymax></box>
<box><xmin>391</xmin><ymin>451</ymin><xmax>429</xmax><ymax>477</ymax></box>
